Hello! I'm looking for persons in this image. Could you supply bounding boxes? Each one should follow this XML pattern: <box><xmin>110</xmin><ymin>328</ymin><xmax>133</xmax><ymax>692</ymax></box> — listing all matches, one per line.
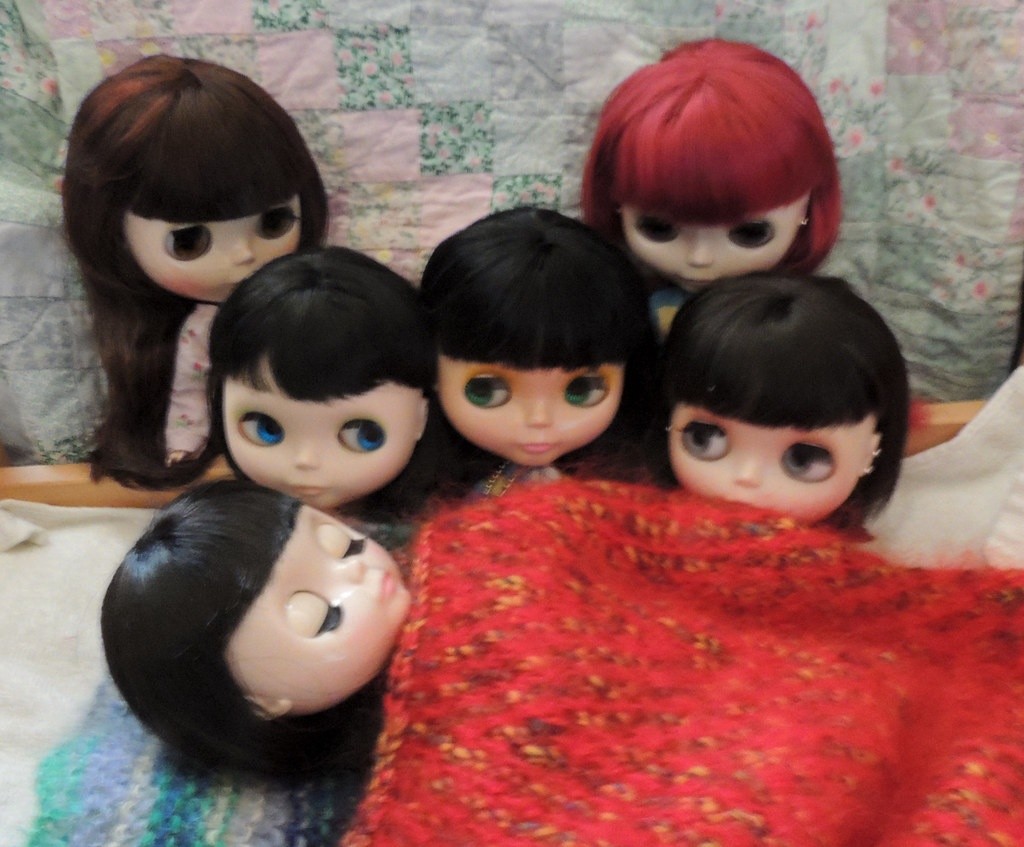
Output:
<box><xmin>100</xmin><ymin>479</ymin><xmax>410</xmax><ymax>773</ymax></box>
<box><xmin>581</xmin><ymin>38</ymin><xmax>844</xmax><ymax>342</ymax></box>
<box><xmin>59</xmin><ymin>58</ymin><xmax>332</xmax><ymax>495</ymax></box>
<box><xmin>204</xmin><ymin>247</ymin><xmax>426</xmax><ymax>524</ymax></box>
<box><xmin>426</xmin><ymin>206</ymin><xmax>655</xmax><ymax>488</ymax></box>
<box><xmin>653</xmin><ymin>271</ymin><xmax>907</xmax><ymax>531</ymax></box>
